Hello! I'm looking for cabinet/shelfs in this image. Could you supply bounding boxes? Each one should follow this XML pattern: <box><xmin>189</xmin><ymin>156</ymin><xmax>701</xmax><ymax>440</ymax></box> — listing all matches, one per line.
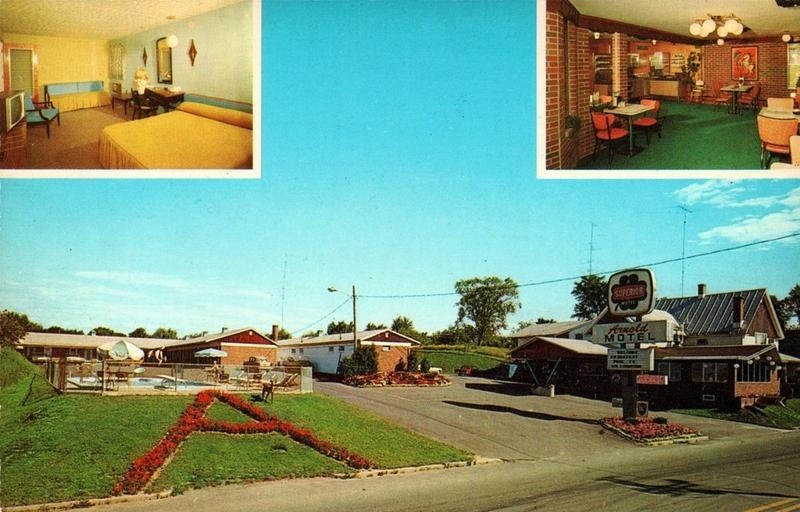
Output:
<box><xmin>0</xmin><ymin>117</ymin><xmax>28</xmax><ymax>169</ymax></box>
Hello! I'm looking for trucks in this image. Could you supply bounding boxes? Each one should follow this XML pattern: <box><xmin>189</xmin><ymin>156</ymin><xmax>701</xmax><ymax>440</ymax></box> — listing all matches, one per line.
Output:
<box><xmin>243</xmin><ymin>356</ymin><xmax>273</xmax><ymax>372</ymax></box>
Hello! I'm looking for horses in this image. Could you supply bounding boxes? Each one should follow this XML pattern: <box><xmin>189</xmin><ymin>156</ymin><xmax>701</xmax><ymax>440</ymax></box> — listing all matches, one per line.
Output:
<box><xmin>741</xmin><ymin>53</ymin><xmax>755</xmax><ymax>76</ymax></box>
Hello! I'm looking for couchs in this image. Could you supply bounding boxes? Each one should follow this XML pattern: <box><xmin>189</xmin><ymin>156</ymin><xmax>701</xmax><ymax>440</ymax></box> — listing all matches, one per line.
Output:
<box><xmin>43</xmin><ymin>79</ymin><xmax>112</xmax><ymax>114</ymax></box>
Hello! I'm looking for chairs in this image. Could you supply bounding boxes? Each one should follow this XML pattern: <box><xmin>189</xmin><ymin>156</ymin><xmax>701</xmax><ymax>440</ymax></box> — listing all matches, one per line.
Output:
<box><xmin>710</xmin><ymin>80</ymin><xmax>800</xmax><ymax>170</ymax></box>
<box><xmin>130</xmin><ymin>90</ymin><xmax>157</xmax><ymax>121</ymax></box>
<box><xmin>24</xmin><ymin>96</ymin><xmax>60</xmax><ymax>138</ymax></box>
<box><xmin>589</xmin><ymin>113</ymin><xmax>630</xmax><ymax>162</ymax></box>
<box><xmin>632</xmin><ymin>99</ymin><xmax>663</xmax><ymax>147</ymax></box>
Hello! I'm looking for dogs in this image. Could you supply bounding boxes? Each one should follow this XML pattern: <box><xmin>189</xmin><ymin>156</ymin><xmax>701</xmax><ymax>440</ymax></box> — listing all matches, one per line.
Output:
<box><xmin>262</xmin><ymin>380</ymin><xmax>274</xmax><ymax>403</ymax></box>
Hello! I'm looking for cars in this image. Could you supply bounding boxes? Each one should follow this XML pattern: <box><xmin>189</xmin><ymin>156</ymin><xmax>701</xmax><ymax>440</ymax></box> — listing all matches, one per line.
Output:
<box><xmin>418</xmin><ymin>364</ymin><xmax>443</xmax><ymax>374</ymax></box>
<box><xmin>28</xmin><ymin>351</ymin><xmax>52</xmax><ymax>364</ymax></box>
<box><xmin>454</xmin><ymin>365</ymin><xmax>487</xmax><ymax>377</ymax></box>
<box><xmin>65</xmin><ymin>353</ymin><xmax>88</xmax><ymax>365</ymax></box>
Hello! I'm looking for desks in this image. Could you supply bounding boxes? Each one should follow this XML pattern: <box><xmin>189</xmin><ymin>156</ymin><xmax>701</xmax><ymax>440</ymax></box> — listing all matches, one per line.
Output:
<box><xmin>145</xmin><ymin>87</ymin><xmax>185</xmax><ymax>112</ymax></box>
<box><xmin>605</xmin><ymin>102</ymin><xmax>655</xmax><ymax>157</ymax></box>
<box><xmin>630</xmin><ymin>79</ymin><xmax>686</xmax><ymax>104</ymax></box>
<box><xmin>111</xmin><ymin>94</ymin><xmax>133</xmax><ymax>114</ymax></box>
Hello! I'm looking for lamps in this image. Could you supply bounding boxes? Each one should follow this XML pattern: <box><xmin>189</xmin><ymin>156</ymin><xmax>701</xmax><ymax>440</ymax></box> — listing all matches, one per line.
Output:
<box><xmin>781</xmin><ymin>30</ymin><xmax>791</xmax><ymax>42</ymax></box>
<box><xmin>688</xmin><ymin>13</ymin><xmax>744</xmax><ymax>46</ymax></box>
<box><xmin>165</xmin><ymin>16</ymin><xmax>179</xmax><ymax>48</ymax></box>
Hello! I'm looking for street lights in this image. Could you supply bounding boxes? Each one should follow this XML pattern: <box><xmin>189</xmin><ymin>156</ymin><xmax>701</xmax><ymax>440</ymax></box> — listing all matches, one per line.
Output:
<box><xmin>328</xmin><ymin>285</ymin><xmax>357</xmax><ymax>354</ymax></box>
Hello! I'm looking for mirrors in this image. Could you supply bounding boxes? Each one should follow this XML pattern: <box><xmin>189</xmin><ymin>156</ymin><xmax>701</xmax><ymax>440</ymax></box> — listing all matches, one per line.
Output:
<box><xmin>154</xmin><ymin>37</ymin><xmax>174</xmax><ymax>85</ymax></box>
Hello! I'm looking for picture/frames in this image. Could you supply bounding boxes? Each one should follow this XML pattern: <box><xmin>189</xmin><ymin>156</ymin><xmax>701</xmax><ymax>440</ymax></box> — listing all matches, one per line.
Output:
<box><xmin>731</xmin><ymin>46</ymin><xmax>758</xmax><ymax>81</ymax></box>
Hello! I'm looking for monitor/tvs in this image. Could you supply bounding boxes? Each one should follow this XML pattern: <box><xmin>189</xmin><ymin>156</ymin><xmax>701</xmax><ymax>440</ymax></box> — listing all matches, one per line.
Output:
<box><xmin>0</xmin><ymin>89</ymin><xmax>26</xmax><ymax>133</ymax></box>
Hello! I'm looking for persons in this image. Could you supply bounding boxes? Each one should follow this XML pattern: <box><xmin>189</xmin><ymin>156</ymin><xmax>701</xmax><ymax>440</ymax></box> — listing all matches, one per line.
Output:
<box><xmin>130</xmin><ymin>68</ymin><xmax>155</xmax><ymax>108</ymax></box>
<box><xmin>258</xmin><ymin>369</ymin><xmax>275</xmax><ymax>402</ymax></box>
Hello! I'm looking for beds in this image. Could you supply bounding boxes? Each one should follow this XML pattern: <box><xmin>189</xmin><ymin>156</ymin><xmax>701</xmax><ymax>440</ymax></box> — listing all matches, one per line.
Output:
<box><xmin>97</xmin><ymin>94</ymin><xmax>253</xmax><ymax>169</ymax></box>
